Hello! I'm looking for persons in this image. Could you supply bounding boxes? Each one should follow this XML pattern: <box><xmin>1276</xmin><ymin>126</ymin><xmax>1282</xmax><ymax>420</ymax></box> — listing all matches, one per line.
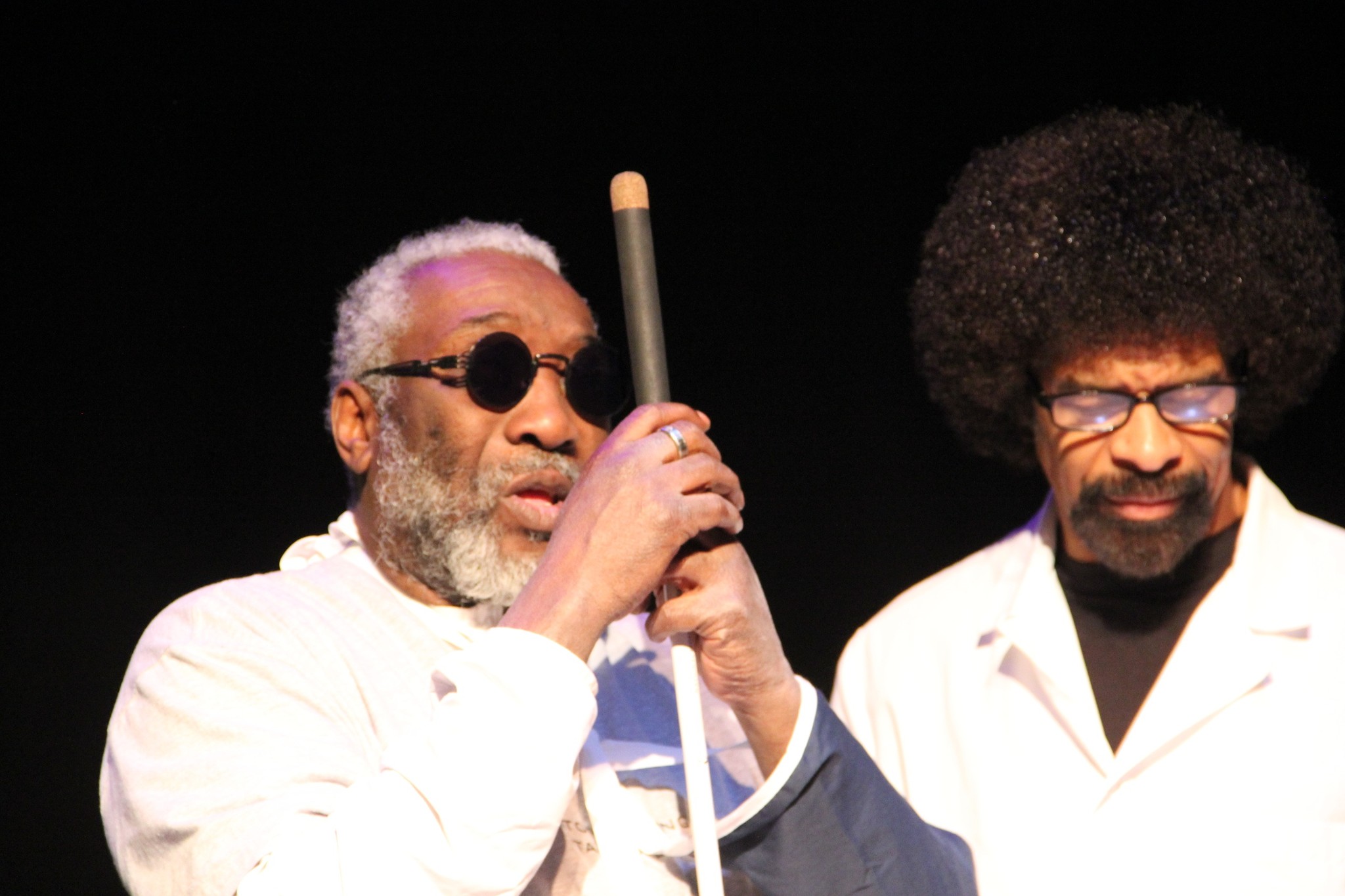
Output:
<box><xmin>100</xmin><ymin>223</ymin><xmax>978</xmax><ymax>896</ymax></box>
<box><xmin>829</xmin><ymin>119</ymin><xmax>1344</xmax><ymax>895</ymax></box>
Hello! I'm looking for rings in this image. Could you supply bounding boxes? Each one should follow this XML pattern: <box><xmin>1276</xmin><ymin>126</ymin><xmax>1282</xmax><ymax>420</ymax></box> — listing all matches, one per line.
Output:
<box><xmin>656</xmin><ymin>426</ymin><xmax>688</xmax><ymax>458</ymax></box>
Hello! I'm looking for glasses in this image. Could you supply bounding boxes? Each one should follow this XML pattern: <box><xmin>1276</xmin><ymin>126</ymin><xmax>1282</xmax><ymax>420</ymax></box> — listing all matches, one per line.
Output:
<box><xmin>1035</xmin><ymin>381</ymin><xmax>1238</xmax><ymax>432</ymax></box>
<box><xmin>356</xmin><ymin>332</ymin><xmax>613</xmax><ymax>433</ymax></box>
